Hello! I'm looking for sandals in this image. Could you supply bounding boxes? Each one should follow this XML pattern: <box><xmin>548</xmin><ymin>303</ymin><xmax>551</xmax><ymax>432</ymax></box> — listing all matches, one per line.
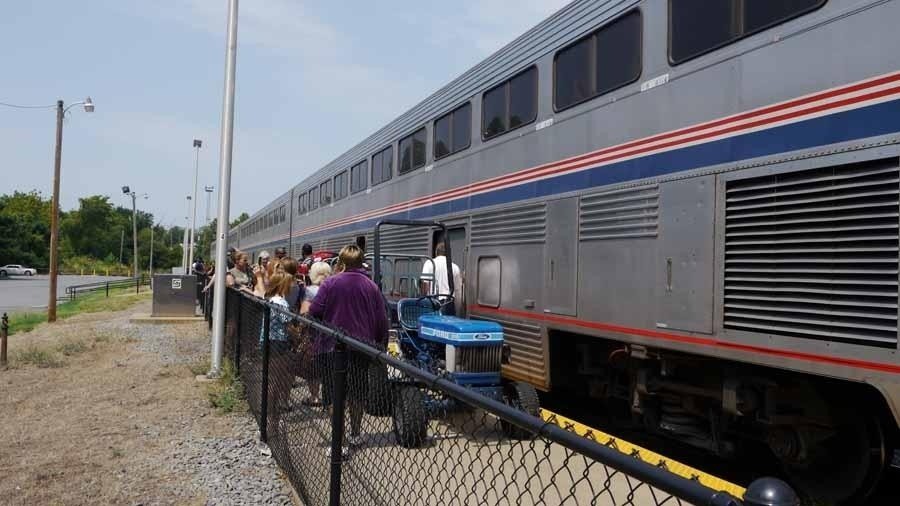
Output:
<box><xmin>302</xmin><ymin>396</ymin><xmax>322</xmax><ymax>407</ymax></box>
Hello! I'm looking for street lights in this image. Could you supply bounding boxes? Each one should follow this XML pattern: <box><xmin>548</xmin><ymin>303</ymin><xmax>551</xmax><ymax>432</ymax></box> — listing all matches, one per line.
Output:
<box><xmin>121</xmin><ymin>186</ymin><xmax>138</xmax><ymax>285</ymax></box>
<box><xmin>47</xmin><ymin>97</ymin><xmax>95</xmax><ymax>322</ymax></box>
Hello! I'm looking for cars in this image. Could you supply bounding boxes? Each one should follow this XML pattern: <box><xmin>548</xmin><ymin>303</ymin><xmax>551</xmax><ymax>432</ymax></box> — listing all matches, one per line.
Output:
<box><xmin>0</xmin><ymin>264</ymin><xmax>37</xmax><ymax>278</ymax></box>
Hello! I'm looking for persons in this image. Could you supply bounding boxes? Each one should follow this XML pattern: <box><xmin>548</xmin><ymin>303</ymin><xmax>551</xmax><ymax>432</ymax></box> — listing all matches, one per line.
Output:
<box><xmin>420</xmin><ymin>241</ymin><xmax>462</xmax><ymax>318</ymax></box>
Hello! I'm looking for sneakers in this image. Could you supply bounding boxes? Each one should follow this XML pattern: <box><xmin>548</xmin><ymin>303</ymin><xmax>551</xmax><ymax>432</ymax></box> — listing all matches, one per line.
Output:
<box><xmin>346</xmin><ymin>430</ymin><xmax>361</xmax><ymax>446</ymax></box>
<box><xmin>319</xmin><ymin>445</ymin><xmax>351</xmax><ymax>459</ymax></box>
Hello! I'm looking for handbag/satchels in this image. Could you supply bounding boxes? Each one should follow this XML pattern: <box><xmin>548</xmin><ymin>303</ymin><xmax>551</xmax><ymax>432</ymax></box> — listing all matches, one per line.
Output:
<box><xmin>287</xmin><ymin>334</ymin><xmax>321</xmax><ymax>379</ymax></box>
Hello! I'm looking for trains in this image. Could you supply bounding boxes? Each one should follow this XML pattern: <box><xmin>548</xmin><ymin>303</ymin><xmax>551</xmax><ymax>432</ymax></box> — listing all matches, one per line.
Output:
<box><xmin>207</xmin><ymin>0</ymin><xmax>900</xmax><ymax>480</ymax></box>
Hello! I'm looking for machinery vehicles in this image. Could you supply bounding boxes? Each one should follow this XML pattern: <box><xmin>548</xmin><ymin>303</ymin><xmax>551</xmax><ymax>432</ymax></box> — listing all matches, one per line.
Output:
<box><xmin>361</xmin><ymin>220</ymin><xmax>542</xmax><ymax>448</ymax></box>
